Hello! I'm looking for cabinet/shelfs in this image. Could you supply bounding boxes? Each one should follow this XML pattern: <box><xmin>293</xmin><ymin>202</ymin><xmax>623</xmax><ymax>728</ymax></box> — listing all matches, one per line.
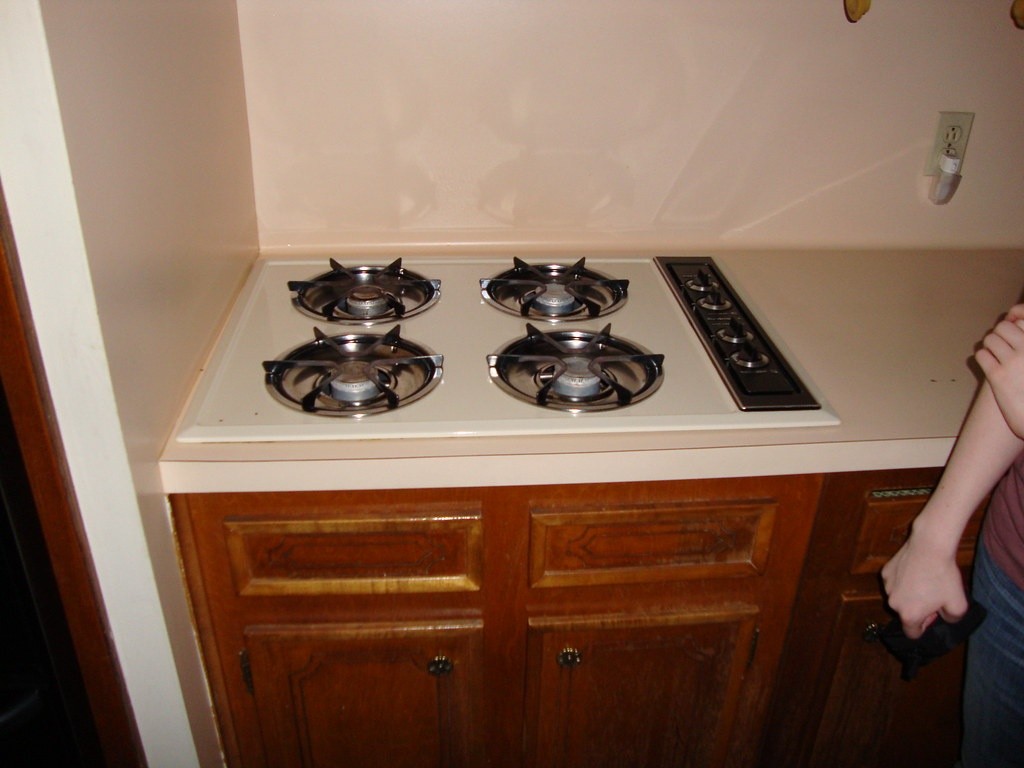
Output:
<box><xmin>167</xmin><ymin>472</ymin><xmax>822</xmax><ymax>768</ymax></box>
<box><xmin>756</xmin><ymin>466</ymin><xmax>990</xmax><ymax>768</ymax></box>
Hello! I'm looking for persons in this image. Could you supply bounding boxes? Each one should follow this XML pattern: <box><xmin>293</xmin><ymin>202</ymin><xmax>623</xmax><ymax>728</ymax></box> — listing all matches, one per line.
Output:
<box><xmin>880</xmin><ymin>296</ymin><xmax>1024</xmax><ymax>768</ymax></box>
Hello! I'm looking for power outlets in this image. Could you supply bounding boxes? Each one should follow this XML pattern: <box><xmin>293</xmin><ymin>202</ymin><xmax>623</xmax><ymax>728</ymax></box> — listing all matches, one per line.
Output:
<box><xmin>923</xmin><ymin>110</ymin><xmax>974</xmax><ymax>176</ymax></box>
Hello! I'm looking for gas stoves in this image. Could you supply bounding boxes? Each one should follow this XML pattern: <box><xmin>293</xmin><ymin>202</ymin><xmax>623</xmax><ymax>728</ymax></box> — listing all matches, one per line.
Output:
<box><xmin>177</xmin><ymin>258</ymin><xmax>841</xmax><ymax>444</ymax></box>
